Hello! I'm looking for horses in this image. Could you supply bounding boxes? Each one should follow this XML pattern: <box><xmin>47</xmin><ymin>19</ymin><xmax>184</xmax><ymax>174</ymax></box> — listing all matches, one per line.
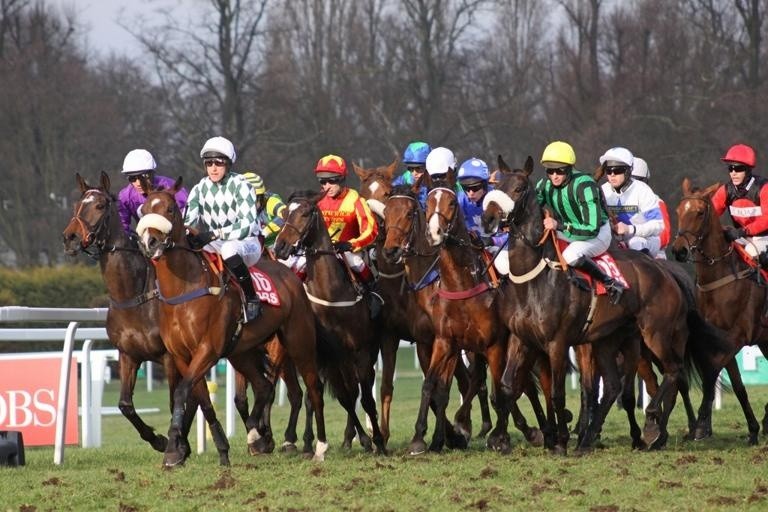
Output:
<box><xmin>60</xmin><ymin>154</ymin><xmax>768</xmax><ymax>472</ymax></box>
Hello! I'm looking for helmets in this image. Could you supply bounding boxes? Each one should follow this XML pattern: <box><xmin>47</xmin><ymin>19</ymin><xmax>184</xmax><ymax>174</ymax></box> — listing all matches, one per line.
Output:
<box><xmin>721</xmin><ymin>143</ymin><xmax>756</xmax><ymax>167</ymax></box>
<box><xmin>122</xmin><ymin>148</ymin><xmax>157</xmax><ymax>174</ymax></box>
<box><xmin>482</xmin><ymin>188</ymin><xmax>515</xmax><ymax>214</ymax></box>
<box><xmin>200</xmin><ymin>136</ymin><xmax>237</xmax><ymax>164</ymax></box>
<box><xmin>540</xmin><ymin>141</ymin><xmax>576</xmax><ymax>165</ymax></box>
<box><xmin>404</xmin><ymin>141</ymin><xmax>432</xmax><ymax>164</ymax></box>
<box><xmin>243</xmin><ymin>172</ymin><xmax>265</xmax><ymax>195</ymax></box>
<box><xmin>599</xmin><ymin>147</ymin><xmax>651</xmax><ymax>178</ymax></box>
<box><xmin>456</xmin><ymin>158</ymin><xmax>490</xmax><ymax>181</ymax></box>
<box><xmin>425</xmin><ymin>146</ymin><xmax>456</xmax><ymax>174</ymax></box>
<box><xmin>315</xmin><ymin>154</ymin><xmax>349</xmax><ymax>177</ymax></box>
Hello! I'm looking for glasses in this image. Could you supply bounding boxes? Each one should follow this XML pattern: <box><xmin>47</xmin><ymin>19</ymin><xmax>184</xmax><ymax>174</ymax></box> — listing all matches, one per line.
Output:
<box><xmin>319</xmin><ymin>178</ymin><xmax>340</xmax><ymax>185</ymax></box>
<box><xmin>431</xmin><ymin>173</ymin><xmax>446</xmax><ymax>179</ymax></box>
<box><xmin>460</xmin><ymin>184</ymin><xmax>484</xmax><ymax>192</ymax></box>
<box><xmin>126</xmin><ymin>173</ymin><xmax>151</xmax><ymax>182</ymax></box>
<box><xmin>204</xmin><ymin>159</ymin><xmax>226</xmax><ymax>166</ymax></box>
<box><xmin>545</xmin><ymin>168</ymin><xmax>569</xmax><ymax>175</ymax></box>
<box><xmin>726</xmin><ymin>164</ymin><xmax>746</xmax><ymax>173</ymax></box>
<box><xmin>406</xmin><ymin>166</ymin><xmax>425</xmax><ymax>173</ymax></box>
<box><xmin>605</xmin><ymin>167</ymin><xmax>626</xmax><ymax>176</ymax></box>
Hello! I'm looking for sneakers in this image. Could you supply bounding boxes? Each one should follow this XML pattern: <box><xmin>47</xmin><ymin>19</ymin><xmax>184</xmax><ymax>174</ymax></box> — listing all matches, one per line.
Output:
<box><xmin>369</xmin><ymin>282</ymin><xmax>385</xmax><ymax>320</ymax></box>
<box><xmin>603</xmin><ymin>279</ymin><xmax>624</xmax><ymax>305</ymax></box>
<box><xmin>243</xmin><ymin>295</ymin><xmax>261</xmax><ymax>322</ymax></box>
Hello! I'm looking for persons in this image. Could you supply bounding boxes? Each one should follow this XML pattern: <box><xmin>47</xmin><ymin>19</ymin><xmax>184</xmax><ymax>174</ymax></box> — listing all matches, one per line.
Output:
<box><xmin>532</xmin><ymin>141</ymin><xmax>672</xmax><ymax>305</ymax></box>
<box><xmin>245</xmin><ymin>136</ymin><xmax>510</xmax><ymax>278</ymax></box>
<box><xmin>117</xmin><ymin>136</ymin><xmax>263</xmax><ymax>320</ymax></box>
<box><xmin>710</xmin><ymin>145</ymin><xmax>768</xmax><ymax>270</ymax></box>
